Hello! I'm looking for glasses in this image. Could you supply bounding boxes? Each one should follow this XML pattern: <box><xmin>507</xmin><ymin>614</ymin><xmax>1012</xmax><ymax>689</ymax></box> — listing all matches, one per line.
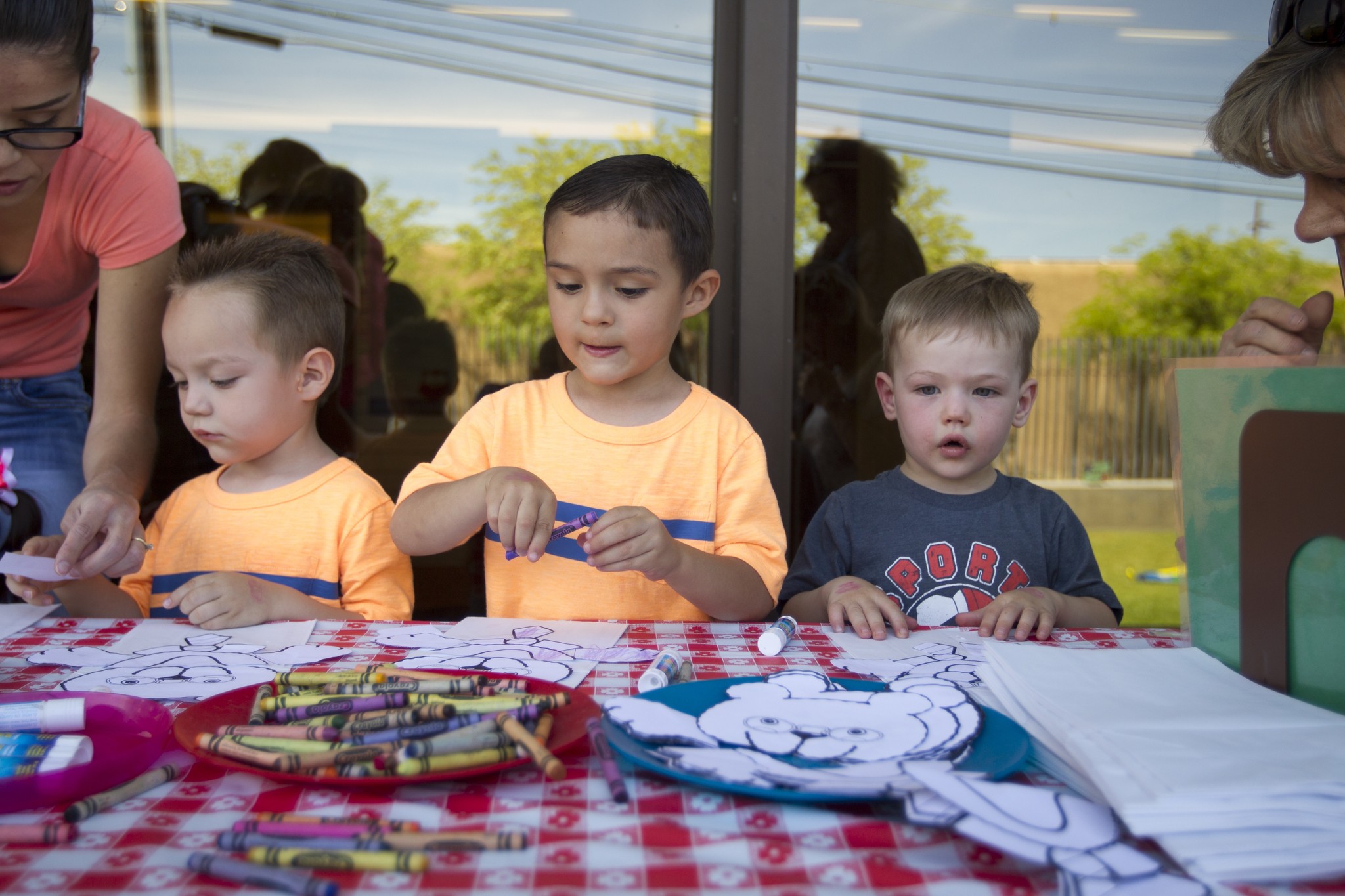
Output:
<box><xmin>0</xmin><ymin>53</ymin><xmax>90</xmax><ymax>151</ymax></box>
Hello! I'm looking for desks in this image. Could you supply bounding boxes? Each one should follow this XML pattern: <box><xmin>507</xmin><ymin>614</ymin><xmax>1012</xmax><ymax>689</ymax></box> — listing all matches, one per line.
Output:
<box><xmin>0</xmin><ymin>616</ymin><xmax>1345</xmax><ymax>896</ymax></box>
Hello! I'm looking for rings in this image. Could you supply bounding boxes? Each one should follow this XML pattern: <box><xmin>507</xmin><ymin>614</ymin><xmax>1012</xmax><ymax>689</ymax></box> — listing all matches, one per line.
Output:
<box><xmin>133</xmin><ymin>536</ymin><xmax>155</xmax><ymax>552</ymax></box>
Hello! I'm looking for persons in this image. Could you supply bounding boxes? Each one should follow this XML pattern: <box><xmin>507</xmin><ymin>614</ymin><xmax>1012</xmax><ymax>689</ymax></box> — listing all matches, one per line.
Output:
<box><xmin>384</xmin><ymin>150</ymin><xmax>791</xmax><ymax>625</ymax></box>
<box><xmin>0</xmin><ymin>220</ymin><xmax>418</xmax><ymax>629</ymax></box>
<box><xmin>1204</xmin><ymin>15</ymin><xmax>1345</xmax><ymax>366</ymax></box>
<box><xmin>772</xmin><ymin>260</ymin><xmax>1122</xmax><ymax>644</ymax></box>
<box><xmin>136</xmin><ymin>116</ymin><xmax>938</xmax><ymax>545</ymax></box>
<box><xmin>0</xmin><ymin>0</ymin><xmax>188</xmax><ymax>607</ymax></box>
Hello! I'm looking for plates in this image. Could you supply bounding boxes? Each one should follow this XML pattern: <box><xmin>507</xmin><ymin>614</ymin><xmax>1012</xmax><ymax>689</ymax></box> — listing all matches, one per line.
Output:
<box><xmin>600</xmin><ymin>675</ymin><xmax>1031</xmax><ymax>801</ymax></box>
<box><xmin>175</xmin><ymin>666</ymin><xmax>599</xmax><ymax>782</ymax></box>
<box><xmin>0</xmin><ymin>686</ymin><xmax>169</xmax><ymax>811</ymax></box>
